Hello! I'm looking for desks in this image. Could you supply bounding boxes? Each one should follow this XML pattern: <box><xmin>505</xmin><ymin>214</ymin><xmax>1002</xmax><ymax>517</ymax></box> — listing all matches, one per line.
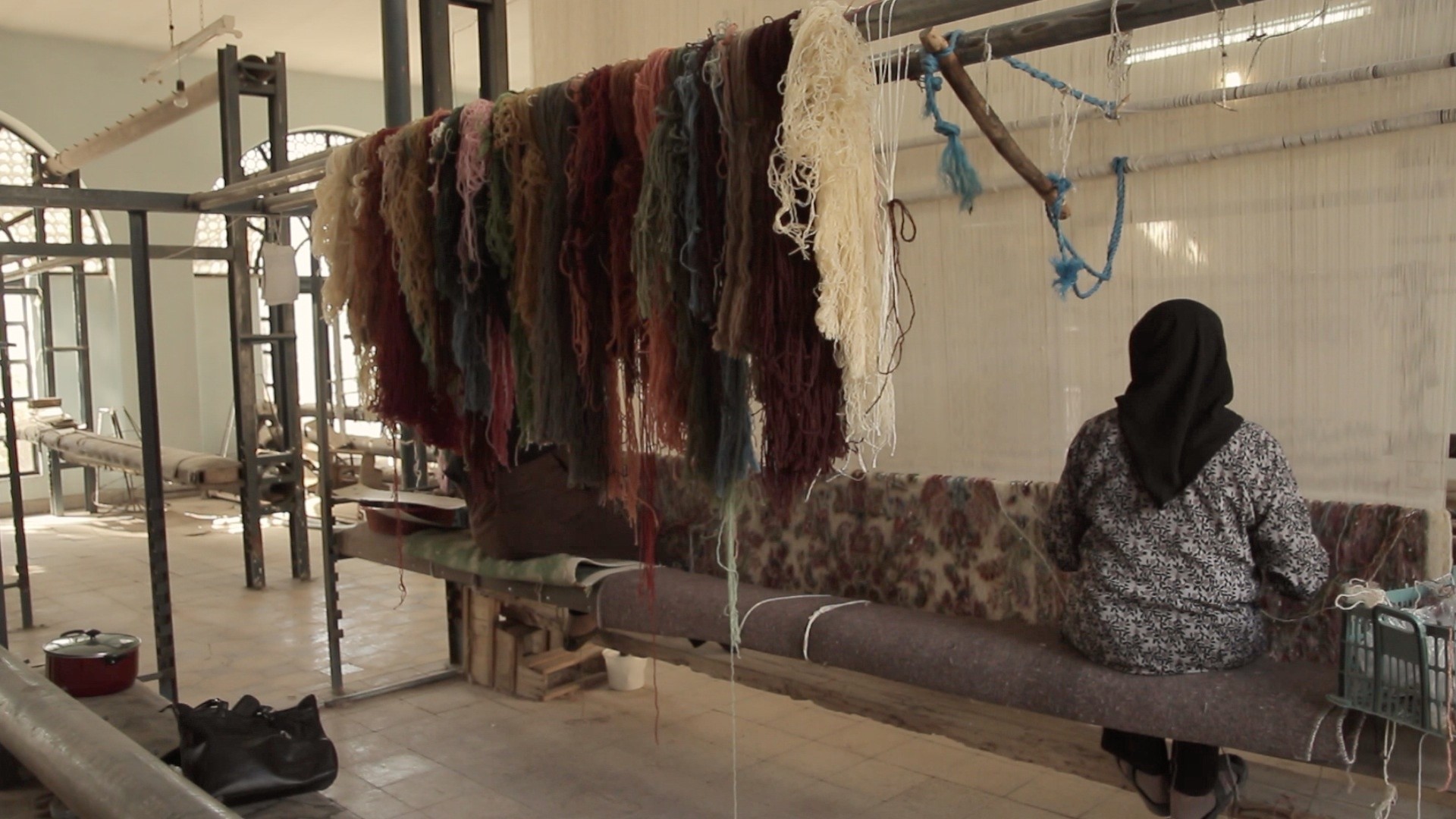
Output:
<box><xmin>0</xmin><ymin>664</ymin><xmax>364</xmax><ymax>819</ymax></box>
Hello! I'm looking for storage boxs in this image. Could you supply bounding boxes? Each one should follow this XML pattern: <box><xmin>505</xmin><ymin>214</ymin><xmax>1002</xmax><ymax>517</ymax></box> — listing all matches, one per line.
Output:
<box><xmin>1325</xmin><ymin>564</ymin><xmax>1456</xmax><ymax>742</ymax></box>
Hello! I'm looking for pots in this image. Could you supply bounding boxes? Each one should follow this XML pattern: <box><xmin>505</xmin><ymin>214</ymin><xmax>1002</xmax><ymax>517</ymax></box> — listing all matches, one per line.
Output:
<box><xmin>42</xmin><ymin>629</ymin><xmax>142</xmax><ymax>698</ymax></box>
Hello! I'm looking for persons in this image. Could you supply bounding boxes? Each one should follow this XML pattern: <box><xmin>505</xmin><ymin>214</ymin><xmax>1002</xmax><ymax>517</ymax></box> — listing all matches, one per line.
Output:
<box><xmin>444</xmin><ymin>408</ymin><xmax>737</xmax><ymax>654</ymax></box>
<box><xmin>1043</xmin><ymin>300</ymin><xmax>1330</xmax><ymax>819</ymax></box>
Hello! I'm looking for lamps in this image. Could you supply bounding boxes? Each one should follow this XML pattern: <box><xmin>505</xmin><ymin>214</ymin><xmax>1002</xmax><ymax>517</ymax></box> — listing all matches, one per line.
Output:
<box><xmin>1123</xmin><ymin>0</ymin><xmax>1381</xmax><ymax>66</ymax></box>
<box><xmin>140</xmin><ymin>0</ymin><xmax>243</xmax><ymax>84</ymax></box>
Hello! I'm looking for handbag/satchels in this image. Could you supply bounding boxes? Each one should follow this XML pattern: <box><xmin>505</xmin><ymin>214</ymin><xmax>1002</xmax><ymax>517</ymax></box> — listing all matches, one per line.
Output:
<box><xmin>166</xmin><ymin>692</ymin><xmax>339</xmax><ymax>807</ymax></box>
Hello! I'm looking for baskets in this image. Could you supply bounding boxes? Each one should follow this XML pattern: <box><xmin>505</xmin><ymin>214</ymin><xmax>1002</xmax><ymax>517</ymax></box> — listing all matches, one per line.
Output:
<box><xmin>1316</xmin><ymin>569</ymin><xmax>1456</xmax><ymax>741</ymax></box>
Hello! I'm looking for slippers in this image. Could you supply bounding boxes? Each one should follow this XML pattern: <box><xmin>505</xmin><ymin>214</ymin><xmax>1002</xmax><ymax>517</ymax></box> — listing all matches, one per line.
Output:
<box><xmin>1115</xmin><ymin>756</ymin><xmax>1170</xmax><ymax>817</ymax></box>
<box><xmin>1200</xmin><ymin>752</ymin><xmax>1249</xmax><ymax>819</ymax></box>
